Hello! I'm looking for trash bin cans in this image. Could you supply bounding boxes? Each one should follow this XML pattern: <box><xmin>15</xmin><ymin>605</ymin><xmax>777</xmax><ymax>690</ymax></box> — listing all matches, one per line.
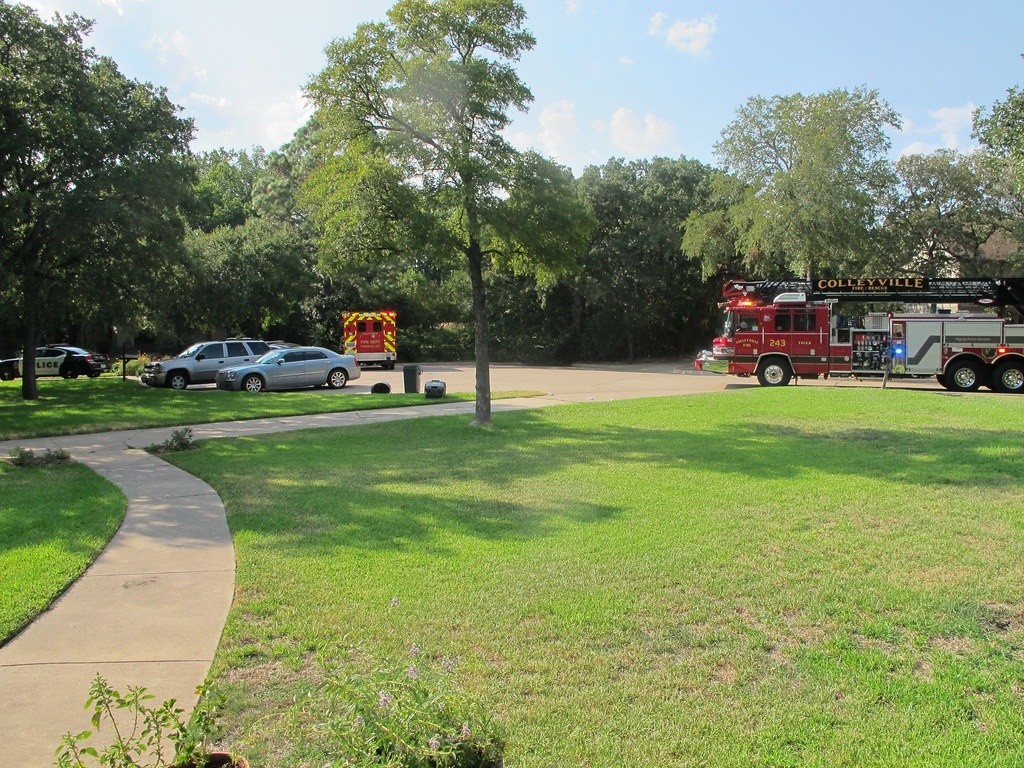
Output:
<box><xmin>403</xmin><ymin>364</ymin><xmax>423</xmax><ymax>393</ymax></box>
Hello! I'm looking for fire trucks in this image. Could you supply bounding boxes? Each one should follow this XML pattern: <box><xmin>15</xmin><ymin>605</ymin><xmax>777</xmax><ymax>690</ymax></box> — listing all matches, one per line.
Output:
<box><xmin>341</xmin><ymin>310</ymin><xmax>396</xmax><ymax>369</ymax></box>
<box><xmin>693</xmin><ymin>276</ymin><xmax>1023</xmax><ymax>394</ymax></box>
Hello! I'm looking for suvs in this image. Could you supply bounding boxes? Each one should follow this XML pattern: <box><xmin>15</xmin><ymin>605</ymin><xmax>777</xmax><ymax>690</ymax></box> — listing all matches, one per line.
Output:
<box><xmin>141</xmin><ymin>339</ymin><xmax>271</xmax><ymax>390</ymax></box>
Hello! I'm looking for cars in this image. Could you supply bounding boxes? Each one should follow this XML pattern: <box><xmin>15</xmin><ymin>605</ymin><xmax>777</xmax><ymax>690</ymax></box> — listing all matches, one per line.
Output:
<box><xmin>0</xmin><ymin>342</ymin><xmax>110</xmax><ymax>382</ymax></box>
<box><xmin>265</xmin><ymin>340</ymin><xmax>301</xmax><ymax>349</ymax></box>
<box><xmin>215</xmin><ymin>346</ymin><xmax>361</xmax><ymax>392</ymax></box>
